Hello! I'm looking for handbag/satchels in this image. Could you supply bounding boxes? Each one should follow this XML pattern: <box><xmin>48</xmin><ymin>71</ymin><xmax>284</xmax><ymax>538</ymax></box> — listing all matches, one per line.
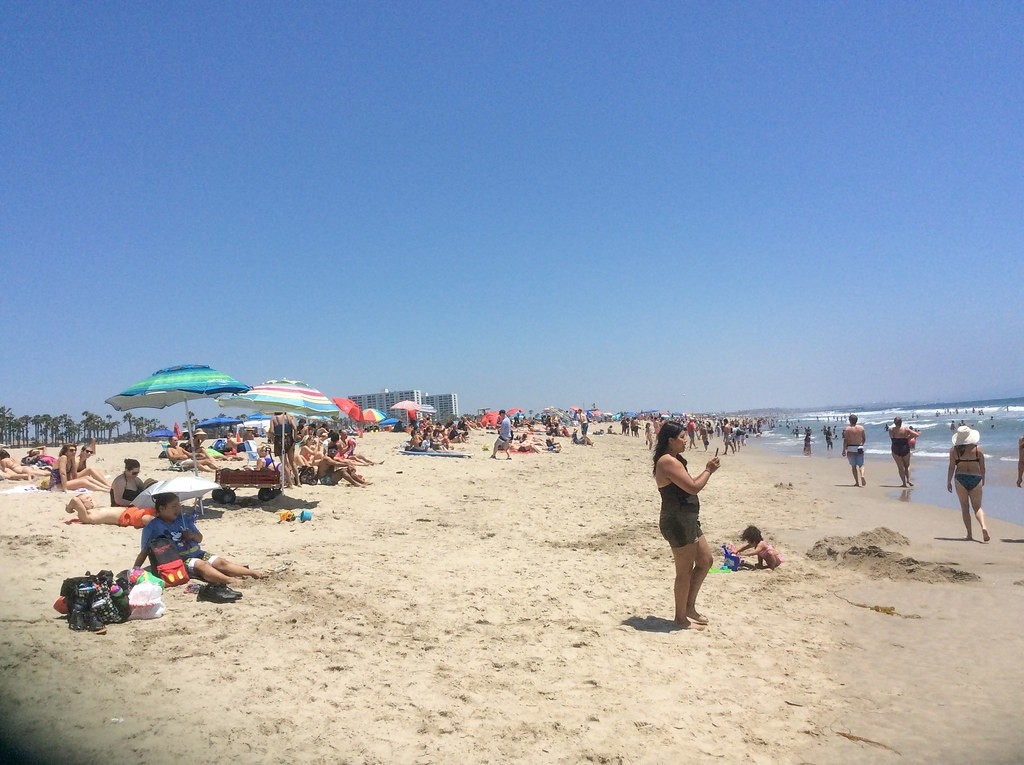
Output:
<box><xmin>704</xmin><ymin>439</ymin><xmax>709</xmax><ymax>445</ymax></box>
<box><xmin>54</xmin><ymin>570</ymin><xmax>166</xmax><ymax>634</ymax></box>
<box><xmin>299</xmin><ymin>466</ymin><xmax>314</xmax><ymax>484</ymax></box>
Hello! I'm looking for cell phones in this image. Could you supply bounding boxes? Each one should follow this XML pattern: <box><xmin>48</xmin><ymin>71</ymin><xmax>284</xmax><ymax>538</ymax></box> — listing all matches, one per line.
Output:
<box><xmin>715</xmin><ymin>448</ymin><xmax>719</xmax><ymax>458</ymax></box>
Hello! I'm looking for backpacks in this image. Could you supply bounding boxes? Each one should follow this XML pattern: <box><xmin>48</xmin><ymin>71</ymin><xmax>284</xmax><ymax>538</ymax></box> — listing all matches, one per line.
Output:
<box><xmin>148</xmin><ymin>537</ymin><xmax>189</xmax><ymax>588</ymax></box>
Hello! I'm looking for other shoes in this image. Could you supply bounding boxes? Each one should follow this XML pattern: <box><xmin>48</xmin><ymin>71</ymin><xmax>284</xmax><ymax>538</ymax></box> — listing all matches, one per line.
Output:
<box><xmin>490</xmin><ymin>455</ymin><xmax>495</xmax><ymax>458</ymax></box>
<box><xmin>507</xmin><ymin>458</ymin><xmax>512</xmax><ymax>459</ymax></box>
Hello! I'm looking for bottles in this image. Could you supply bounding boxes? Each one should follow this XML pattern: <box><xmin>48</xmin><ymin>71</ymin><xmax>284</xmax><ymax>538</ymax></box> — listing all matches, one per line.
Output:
<box><xmin>109</xmin><ymin>585</ymin><xmax>123</xmax><ymax>597</ymax></box>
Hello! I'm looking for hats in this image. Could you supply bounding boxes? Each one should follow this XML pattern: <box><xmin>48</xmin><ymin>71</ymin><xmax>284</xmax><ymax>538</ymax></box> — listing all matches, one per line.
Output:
<box><xmin>143</xmin><ymin>478</ymin><xmax>159</xmax><ymax>489</ymax></box>
<box><xmin>193</xmin><ymin>428</ymin><xmax>207</xmax><ymax>435</ymax></box>
<box><xmin>499</xmin><ymin>410</ymin><xmax>505</xmax><ymax>413</ymax></box>
<box><xmin>952</xmin><ymin>426</ymin><xmax>980</xmax><ymax>445</ymax></box>
<box><xmin>244</xmin><ymin>426</ymin><xmax>254</xmax><ymax>430</ymax></box>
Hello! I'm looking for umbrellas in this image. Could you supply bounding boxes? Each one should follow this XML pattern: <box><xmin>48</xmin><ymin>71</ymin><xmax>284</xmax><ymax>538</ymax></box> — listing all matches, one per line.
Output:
<box><xmin>482</xmin><ymin>411</ymin><xmax>500</xmax><ymax>426</ymax></box>
<box><xmin>104</xmin><ymin>364</ymin><xmax>250</xmax><ymax>516</ymax></box>
<box><xmin>362</xmin><ymin>400</ymin><xmax>437</xmax><ymax>424</ymax></box>
<box><xmin>570</xmin><ymin>404</ymin><xmax>716</xmax><ymax>421</ymax></box>
<box><xmin>506</xmin><ymin>408</ymin><xmax>523</xmax><ymax>416</ymax></box>
<box><xmin>333</xmin><ymin>397</ymin><xmax>364</xmax><ymax>423</ymax></box>
<box><xmin>145</xmin><ymin>412</ymin><xmax>272</xmax><ymax>438</ymax></box>
<box><xmin>214</xmin><ymin>378</ymin><xmax>340</xmax><ymax>496</ymax></box>
<box><xmin>130</xmin><ymin>477</ymin><xmax>223</xmax><ymax>530</ymax></box>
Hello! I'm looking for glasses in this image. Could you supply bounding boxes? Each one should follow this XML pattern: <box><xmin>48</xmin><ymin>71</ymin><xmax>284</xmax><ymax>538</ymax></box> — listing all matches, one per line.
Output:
<box><xmin>84</xmin><ymin>450</ymin><xmax>93</xmax><ymax>454</ymax></box>
<box><xmin>129</xmin><ymin>470</ymin><xmax>139</xmax><ymax>475</ymax></box>
<box><xmin>67</xmin><ymin>449</ymin><xmax>77</xmax><ymax>452</ymax></box>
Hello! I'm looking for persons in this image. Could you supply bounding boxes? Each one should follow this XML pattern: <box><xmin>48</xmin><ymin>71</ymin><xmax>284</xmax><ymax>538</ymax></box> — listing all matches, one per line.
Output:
<box><xmin>842</xmin><ymin>414</ymin><xmax>866</xmax><ymax>486</ymax></box>
<box><xmin>947</xmin><ymin>425</ymin><xmax>991</xmax><ymax>542</ymax></box>
<box><xmin>394</xmin><ymin>406</ymin><xmax>995</xmax><ymax>460</ymax></box>
<box><xmin>705</xmin><ymin>470</ymin><xmax>711</xmax><ymax>475</ymax></box>
<box><xmin>0</xmin><ymin>412</ymin><xmax>385</xmax><ymax>585</ymax></box>
<box><xmin>216</xmin><ymin>426</ymin><xmax>221</xmax><ymax>438</ymax></box>
<box><xmin>730</xmin><ymin>525</ymin><xmax>784</xmax><ymax>570</ymax></box>
<box><xmin>1016</xmin><ymin>436</ymin><xmax>1024</xmax><ymax>488</ymax></box>
<box><xmin>889</xmin><ymin>416</ymin><xmax>920</xmax><ymax>487</ymax></box>
<box><xmin>653</xmin><ymin>420</ymin><xmax>720</xmax><ymax>630</ymax></box>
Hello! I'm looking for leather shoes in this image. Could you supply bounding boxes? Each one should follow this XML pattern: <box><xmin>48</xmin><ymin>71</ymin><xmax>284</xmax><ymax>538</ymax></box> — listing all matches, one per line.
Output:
<box><xmin>197</xmin><ymin>585</ymin><xmax>235</xmax><ymax>604</ymax></box>
<box><xmin>212</xmin><ymin>583</ymin><xmax>242</xmax><ymax>597</ymax></box>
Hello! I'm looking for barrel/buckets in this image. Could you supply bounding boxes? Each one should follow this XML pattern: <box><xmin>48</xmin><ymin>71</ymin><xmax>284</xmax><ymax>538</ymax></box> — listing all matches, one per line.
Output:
<box><xmin>295</xmin><ymin>510</ymin><xmax>312</xmax><ymax>522</ymax></box>
<box><xmin>723</xmin><ymin>553</ymin><xmax>740</xmax><ymax>571</ymax></box>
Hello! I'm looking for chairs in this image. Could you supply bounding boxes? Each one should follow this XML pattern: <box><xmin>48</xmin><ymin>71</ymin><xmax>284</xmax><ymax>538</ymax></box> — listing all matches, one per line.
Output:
<box><xmin>243</xmin><ymin>440</ymin><xmax>260</xmax><ymax>465</ymax></box>
<box><xmin>159</xmin><ymin>440</ymin><xmax>191</xmax><ymax>473</ymax></box>
